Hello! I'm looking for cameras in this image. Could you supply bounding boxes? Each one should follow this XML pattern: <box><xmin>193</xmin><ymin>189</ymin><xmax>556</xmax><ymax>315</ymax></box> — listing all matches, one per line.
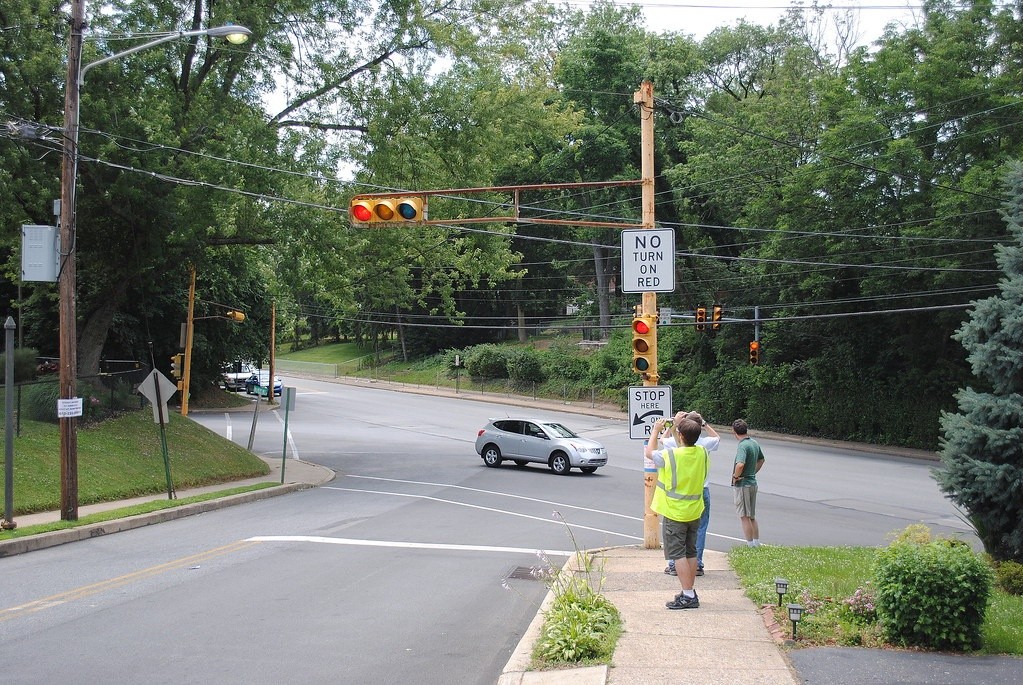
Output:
<box><xmin>663</xmin><ymin>418</ymin><xmax>673</xmax><ymax>428</ymax></box>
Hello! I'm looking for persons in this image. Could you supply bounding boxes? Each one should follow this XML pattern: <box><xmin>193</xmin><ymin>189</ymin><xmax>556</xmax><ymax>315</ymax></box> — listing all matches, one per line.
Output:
<box><xmin>659</xmin><ymin>412</ymin><xmax>721</xmax><ymax>576</ymax></box>
<box><xmin>731</xmin><ymin>419</ymin><xmax>765</xmax><ymax>548</ymax></box>
<box><xmin>646</xmin><ymin>417</ymin><xmax>710</xmax><ymax>609</ymax></box>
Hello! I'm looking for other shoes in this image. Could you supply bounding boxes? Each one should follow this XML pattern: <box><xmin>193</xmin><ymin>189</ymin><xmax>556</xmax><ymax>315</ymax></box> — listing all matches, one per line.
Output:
<box><xmin>666</xmin><ymin>592</ymin><xmax>698</xmax><ymax>609</ymax></box>
<box><xmin>675</xmin><ymin>589</ymin><xmax>700</xmax><ymax>606</ymax></box>
<box><xmin>695</xmin><ymin>565</ymin><xmax>705</xmax><ymax>576</ymax></box>
<box><xmin>664</xmin><ymin>566</ymin><xmax>678</xmax><ymax>576</ymax></box>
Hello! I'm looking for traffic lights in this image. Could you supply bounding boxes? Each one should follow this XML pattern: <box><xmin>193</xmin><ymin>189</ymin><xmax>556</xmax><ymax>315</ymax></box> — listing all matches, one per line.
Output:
<box><xmin>351</xmin><ymin>197</ymin><xmax>423</xmax><ymax>223</ymax></box>
<box><xmin>177</xmin><ymin>381</ymin><xmax>182</xmax><ymax>390</ymax></box>
<box><xmin>170</xmin><ymin>356</ymin><xmax>181</xmax><ymax>378</ymax></box>
<box><xmin>632</xmin><ymin>317</ymin><xmax>657</xmax><ymax>374</ymax></box>
<box><xmin>750</xmin><ymin>341</ymin><xmax>758</xmax><ymax>364</ymax></box>
<box><xmin>713</xmin><ymin>307</ymin><xmax>722</xmax><ymax>329</ymax></box>
<box><xmin>697</xmin><ymin>308</ymin><xmax>705</xmax><ymax>331</ymax></box>
<box><xmin>227</xmin><ymin>311</ymin><xmax>245</xmax><ymax>321</ymax></box>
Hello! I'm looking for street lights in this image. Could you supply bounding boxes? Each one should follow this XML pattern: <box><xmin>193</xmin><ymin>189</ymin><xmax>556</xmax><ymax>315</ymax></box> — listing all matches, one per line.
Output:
<box><xmin>59</xmin><ymin>26</ymin><xmax>252</xmax><ymax>522</ymax></box>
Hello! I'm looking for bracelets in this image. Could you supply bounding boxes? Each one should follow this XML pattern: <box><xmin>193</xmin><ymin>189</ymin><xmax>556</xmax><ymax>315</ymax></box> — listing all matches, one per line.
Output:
<box><xmin>733</xmin><ymin>476</ymin><xmax>738</xmax><ymax>479</ymax></box>
<box><xmin>702</xmin><ymin>422</ymin><xmax>707</xmax><ymax>427</ymax></box>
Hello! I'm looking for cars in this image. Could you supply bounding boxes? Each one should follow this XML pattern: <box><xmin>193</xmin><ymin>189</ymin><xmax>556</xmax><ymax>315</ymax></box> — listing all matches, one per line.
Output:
<box><xmin>475</xmin><ymin>418</ymin><xmax>608</xmax><ymax>475</ymax></box>
<box><xmin>245</xmin><ymin>371</ymin><xmax>283</xmax><ymax>396</ymax></box>
<box><xmin>211</xmin><ymin>354</ymin><xmax>258</xmax><ymax>392</ymax></box>
